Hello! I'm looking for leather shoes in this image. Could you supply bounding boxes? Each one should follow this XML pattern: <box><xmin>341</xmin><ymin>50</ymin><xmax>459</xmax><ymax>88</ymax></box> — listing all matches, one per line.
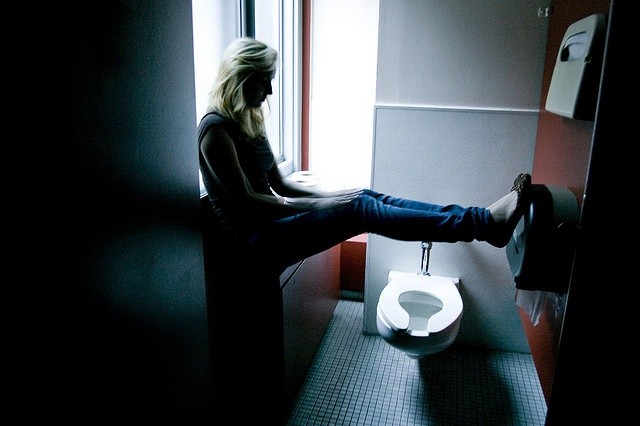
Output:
<box><xmin>491</xmin><ymin>173</ymin><xmax>531</xmax><ymax>249</ymax></box>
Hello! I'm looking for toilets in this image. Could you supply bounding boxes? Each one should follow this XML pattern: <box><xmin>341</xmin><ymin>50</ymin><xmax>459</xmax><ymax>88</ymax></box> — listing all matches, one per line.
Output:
<box><xmin>376</xmin><ymin>270</ymin><xmax>464</xmax><ymax>359</ymax></box>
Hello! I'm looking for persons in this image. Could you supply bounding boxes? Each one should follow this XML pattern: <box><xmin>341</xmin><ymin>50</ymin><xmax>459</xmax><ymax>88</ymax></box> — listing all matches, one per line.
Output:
<box><xmin>196</xmin><ymin>37</ymin><xmax>532</xmax><ymax>269</ymax></box>
